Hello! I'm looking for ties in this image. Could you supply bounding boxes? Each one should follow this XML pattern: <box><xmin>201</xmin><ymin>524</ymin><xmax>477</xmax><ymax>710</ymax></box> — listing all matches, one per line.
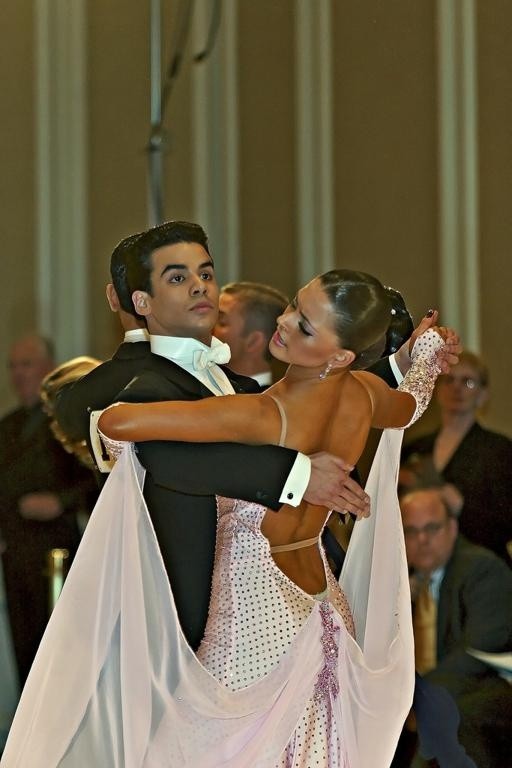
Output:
<box><xmin>407</xmin><ymin>574</ymin><xmax>440</xmax><ymax>678</ymax></box>
<box><xmin>192</xmin><ymin>343</ymin><xmax>240</xmax><ymax>396</ymax></box>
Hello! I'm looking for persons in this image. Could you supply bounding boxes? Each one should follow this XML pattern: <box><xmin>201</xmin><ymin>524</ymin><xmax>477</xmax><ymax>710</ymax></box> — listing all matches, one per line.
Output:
<box><xmin>57</xmin><ymin>229</ymin><xmax>151</xmax><ymax>441</ymax></box>
<box><xmin>214</xmin><ymin>283</ymin><xmax>290</xmax><ymax>388</ymax></box>
<box><xmin>400</xmin><ymin>352</ymin><xmax>506</xmax><ymax>568</ymax></box>
<box><xmin>388</xmin><ymin>481</ymin><xmax>511</xmax><ymax>767</ymax></box>
<box><xmin>0</xmin><ymin>338</ymin><xmax>99</xmax><ymax>686</ymax></box>
